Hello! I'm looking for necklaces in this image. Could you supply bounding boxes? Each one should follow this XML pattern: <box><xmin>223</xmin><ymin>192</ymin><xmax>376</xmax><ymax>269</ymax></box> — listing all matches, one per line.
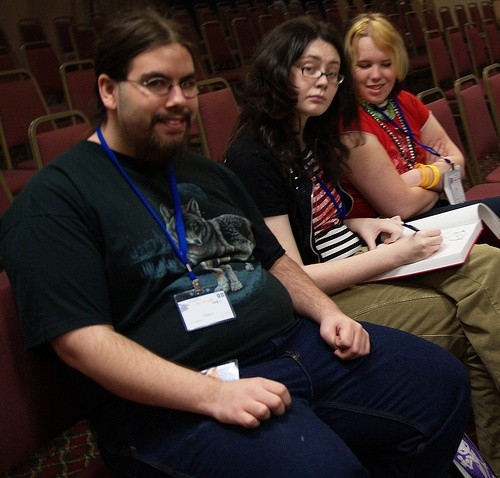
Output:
<box><xmin>357</xmin><ymin>98</ymin><xmax>417</xmax><ymax>171</ymax></box>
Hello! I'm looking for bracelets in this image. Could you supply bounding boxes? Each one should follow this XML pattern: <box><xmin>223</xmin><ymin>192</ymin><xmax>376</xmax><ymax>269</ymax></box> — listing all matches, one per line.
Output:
<box><xmin>413</xmin><ymin>162</ymin><xmax>428</xmax><ymax>188</ymax></box>
<box><xmin>425</xmin><ymin>165</ymin><xmax>440</xmax><ymax>190</ymax></box>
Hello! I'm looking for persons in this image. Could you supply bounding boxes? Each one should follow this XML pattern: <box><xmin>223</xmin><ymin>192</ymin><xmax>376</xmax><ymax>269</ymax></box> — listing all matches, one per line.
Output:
<box><xmin>0</xmin><ymin>7</ymin><xmax>479</xmax><ymax>478</ymax></box>
<box><xmin>221</xmin><ymin>16</ymin><xmax>500</xmax><ymax>478</ymax></box>
<box><xmin>332</xmin><ymin>13</ymin><xmax>499</xmax><ymax>222</ymax></box>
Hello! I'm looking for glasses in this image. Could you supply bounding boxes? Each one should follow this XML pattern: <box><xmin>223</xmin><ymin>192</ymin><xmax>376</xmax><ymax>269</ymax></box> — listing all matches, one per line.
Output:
<box><xmin>126</xmin><ymin>78</ymin><xmax>203</xmax><ymax>98</ymax></box>
<box><xmin>294</xmin><ymin>63</ymin><xmax>345</xmax><ymax>85</ymax></box>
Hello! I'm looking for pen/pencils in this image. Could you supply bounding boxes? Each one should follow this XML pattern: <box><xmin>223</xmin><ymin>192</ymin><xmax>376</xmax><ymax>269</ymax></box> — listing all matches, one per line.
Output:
<box><xmin>400</xmin><ymin>222</ymin><xmax>422</xmax><ymax>232</ymax></box>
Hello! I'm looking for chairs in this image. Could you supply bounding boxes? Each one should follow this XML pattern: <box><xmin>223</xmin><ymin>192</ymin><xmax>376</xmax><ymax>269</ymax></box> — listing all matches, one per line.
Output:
<box><xmin>0</xmin><ymin>0</ymin><xmax>500</xmax><ymax>221</ymax></box>
<box><xmin>0</xmin><ymin>270</ymin><xmax>105</xmax><ymax>478</ymax></box>
